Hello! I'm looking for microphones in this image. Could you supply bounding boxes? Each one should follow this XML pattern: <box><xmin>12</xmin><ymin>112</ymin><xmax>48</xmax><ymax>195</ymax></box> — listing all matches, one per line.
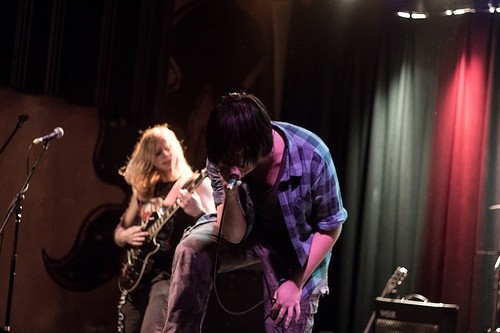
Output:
<box><xmin>226</xmin><ymin>175</ymin><xmax>238</xmax><ymax>190</ymax></box>
<box><xmin>33</xmin><ymin>127</ymin><xmax>64</xmax><ymax>144</ymax></box>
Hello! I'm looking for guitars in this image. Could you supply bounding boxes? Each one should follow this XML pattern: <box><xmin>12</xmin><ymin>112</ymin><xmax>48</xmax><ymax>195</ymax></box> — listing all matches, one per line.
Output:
<box><xmin>116</xmin><ymin>158</ymin><xmax>210</xmax><ymax>294</ymax></box>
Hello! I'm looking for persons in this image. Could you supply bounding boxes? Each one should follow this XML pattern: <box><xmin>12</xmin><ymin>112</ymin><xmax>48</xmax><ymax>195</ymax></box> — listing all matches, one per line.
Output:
<box><xmin>162</xmin><ymin>93</ymin><xmax>349</xmax><ymax>333</ymax></box>
<box><xmin>111</xmin><ymin>124</ymin><xmax>218</xmax><ymax>333</ymax></box>
